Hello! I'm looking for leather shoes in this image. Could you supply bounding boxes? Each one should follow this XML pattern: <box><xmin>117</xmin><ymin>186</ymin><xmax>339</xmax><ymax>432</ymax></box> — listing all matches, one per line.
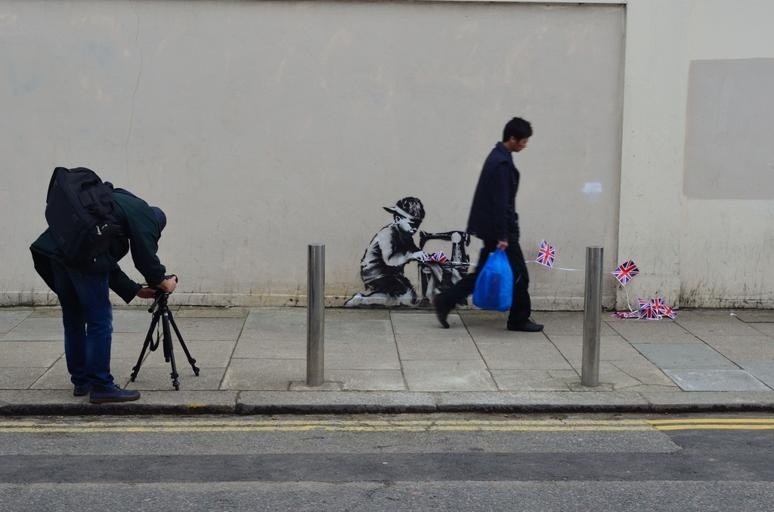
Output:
<box><xmin>506</xmin><ymin>317</ymin><xmax>544</xmax><ymax>332</ymax></box>
<box><xmin>73</xmin><ymin>381</ymin><xmax>140</xmax><ymax>404</ymax></box>
<box><xmin>433</xmin><ymin>296</ymin><xmax>450</xmax><ymax>328</ymax></box>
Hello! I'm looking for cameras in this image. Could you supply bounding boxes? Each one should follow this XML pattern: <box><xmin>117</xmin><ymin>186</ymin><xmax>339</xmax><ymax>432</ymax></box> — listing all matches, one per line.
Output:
<box><xmin>157</xmin><ymin>273</ymin><xmax>178</xmax><ymax>295</ymax></box>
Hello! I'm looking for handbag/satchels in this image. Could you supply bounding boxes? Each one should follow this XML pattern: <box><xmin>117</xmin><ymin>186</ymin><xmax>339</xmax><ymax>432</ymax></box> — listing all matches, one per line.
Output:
<box><xmin>63</xmin><ymin>210</ymin><xmax>100</xmax><ymax>264</ymax></box>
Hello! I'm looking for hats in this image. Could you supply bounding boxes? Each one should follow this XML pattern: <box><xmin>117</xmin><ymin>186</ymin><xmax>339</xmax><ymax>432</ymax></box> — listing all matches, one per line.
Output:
<box><xmin>382</xmin><ymin>196</ymin><xmax>425</xmax><ymax>221</ymax></box>
<box><xmin>149</xmin><ymin>206</ymin><xmax>166</xmax><ymax>230</ymax></box>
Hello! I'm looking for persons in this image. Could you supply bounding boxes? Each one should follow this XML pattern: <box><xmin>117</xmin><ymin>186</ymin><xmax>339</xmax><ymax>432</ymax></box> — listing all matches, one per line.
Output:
<box><xmin>30</xmin><ymin>188</ymin><xmax>177</xmax><ymax>403</ymax></box>
<box><xmin>344</xmin><ymin>197</ymin><xmax>429</xmax><ymax>308</ymax></box>
<box><xmin>433</xmin><ymin>117</ymin><xmax>544</xmax><ymax>332</ymax></box>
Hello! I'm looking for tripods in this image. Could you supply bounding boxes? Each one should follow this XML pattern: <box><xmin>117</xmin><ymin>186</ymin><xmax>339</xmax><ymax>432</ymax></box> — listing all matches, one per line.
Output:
<box><xmin>129</xmin><ymin>292</ymin><xmax>200</xmax><ymax>391</ymax></box>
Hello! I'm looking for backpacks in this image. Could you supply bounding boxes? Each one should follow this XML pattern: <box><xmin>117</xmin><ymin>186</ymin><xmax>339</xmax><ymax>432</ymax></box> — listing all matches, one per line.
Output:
<box><xmin>45</xmin><ymin>166</ymin><xmax>114</xmax><ymax>224</ymax></box>
